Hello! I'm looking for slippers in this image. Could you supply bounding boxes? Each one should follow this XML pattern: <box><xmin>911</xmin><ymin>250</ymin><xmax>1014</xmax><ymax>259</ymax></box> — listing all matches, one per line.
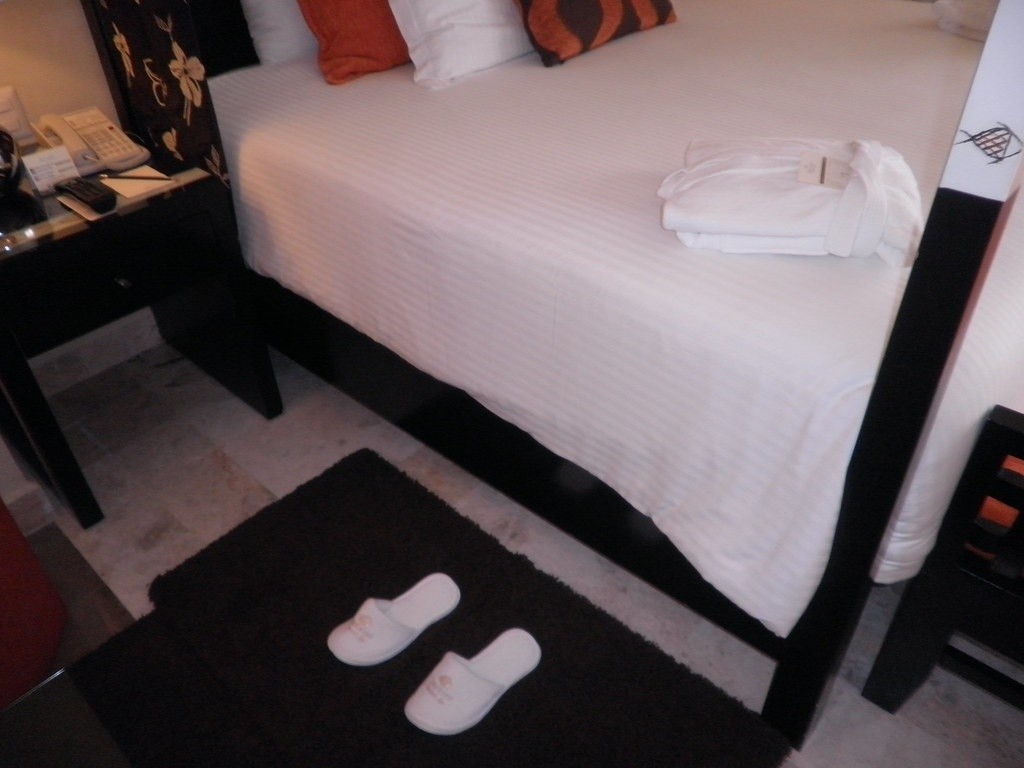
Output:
<box><xmin>327</xmin><ymin>573</ymin><xmax>461</xmax><ymax>666</ymax></box>
<box><xmin>404</xmin><ymin>627</ymin><xmax>541</xmax><ymax>735</ymax></box>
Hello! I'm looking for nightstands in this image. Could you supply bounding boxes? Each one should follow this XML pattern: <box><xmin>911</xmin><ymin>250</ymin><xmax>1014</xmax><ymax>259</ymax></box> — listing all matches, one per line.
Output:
<box><xmin>0</xmin><ymin>132</ymin><xmax>285</xmax><ymax>532</ymax></box>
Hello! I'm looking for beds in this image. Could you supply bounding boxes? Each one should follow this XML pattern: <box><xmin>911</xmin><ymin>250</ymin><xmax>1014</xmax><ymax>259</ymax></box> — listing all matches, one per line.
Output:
<box><xmin>80</xmin><ymin>0</ymin><xmax>1024</xmax><ymax>754</ymax></box>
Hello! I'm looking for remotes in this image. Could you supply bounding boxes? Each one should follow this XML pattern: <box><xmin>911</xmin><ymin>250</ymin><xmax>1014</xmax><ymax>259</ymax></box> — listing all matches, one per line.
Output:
<box><xmin>53</xmin><ymin>176</ymin><xmax>117</xmax><ymax>214</ymax></box>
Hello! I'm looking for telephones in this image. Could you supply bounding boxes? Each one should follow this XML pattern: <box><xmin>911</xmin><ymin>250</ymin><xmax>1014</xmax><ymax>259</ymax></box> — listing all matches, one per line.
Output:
<box><xmin>28</xmin><ymin>105</ymin><xmax>151</xmax><ymax>177</ymax></box>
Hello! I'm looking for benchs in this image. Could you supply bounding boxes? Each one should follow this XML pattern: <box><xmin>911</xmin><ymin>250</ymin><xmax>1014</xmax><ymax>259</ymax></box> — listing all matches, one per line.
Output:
<box><xmin>859</xmin><ymin>404</ymin><xmax>1024</xmax><ymax>714</ymax></box>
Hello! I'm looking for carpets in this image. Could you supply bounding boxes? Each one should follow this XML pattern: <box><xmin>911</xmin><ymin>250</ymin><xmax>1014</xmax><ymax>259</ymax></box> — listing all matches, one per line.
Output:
<box><xmin>154</xmin><ymin>448</ymin><xmax>795</xmax><ymax>768</ymax></box>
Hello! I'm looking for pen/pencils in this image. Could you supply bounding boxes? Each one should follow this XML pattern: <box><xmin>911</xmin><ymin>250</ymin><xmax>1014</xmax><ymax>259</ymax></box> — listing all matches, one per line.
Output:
<box><xmin>100</xmin><ymin>174</ymin><xmax>171</xmax><ymax>180</ymax></box>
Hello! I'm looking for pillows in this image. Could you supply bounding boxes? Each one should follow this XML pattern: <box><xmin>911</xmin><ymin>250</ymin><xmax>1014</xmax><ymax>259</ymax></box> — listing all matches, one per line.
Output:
<box><xmin>239</xmin><ymin>0</ymin><xmax>679</xmax><ymax>91</ymax></box>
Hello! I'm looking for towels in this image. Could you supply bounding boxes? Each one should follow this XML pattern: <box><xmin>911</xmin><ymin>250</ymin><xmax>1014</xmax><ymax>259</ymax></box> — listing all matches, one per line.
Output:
<box><xmin>657</xmin><ymin>138</ymin><xmax>925</xmax><ymax>268</ymax></box>
